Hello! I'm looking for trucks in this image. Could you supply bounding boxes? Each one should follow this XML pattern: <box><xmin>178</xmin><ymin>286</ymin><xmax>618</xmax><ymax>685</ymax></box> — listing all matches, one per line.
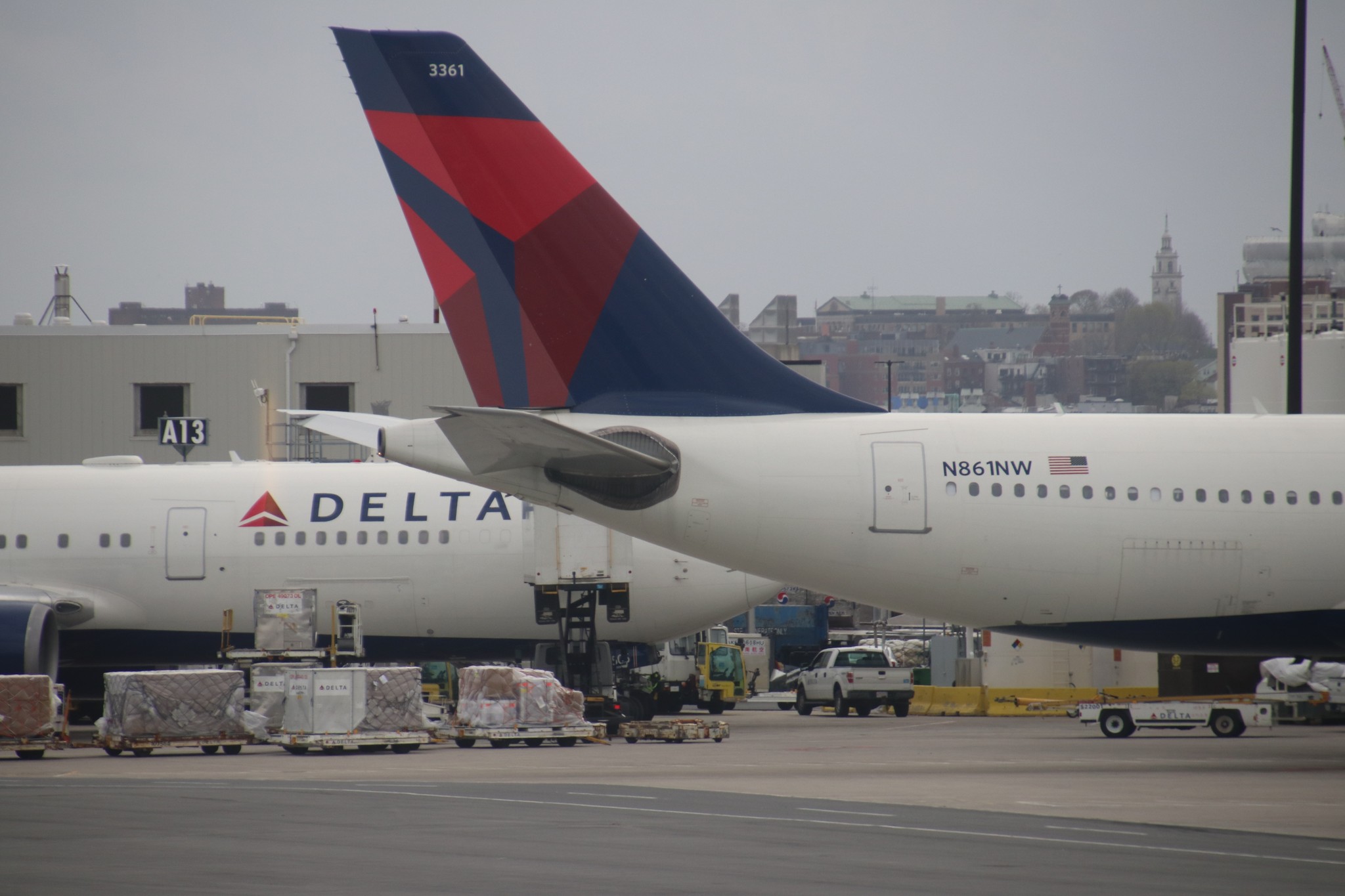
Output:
<box><xmin>1079</xmin><ymin>703</ymin><xmax>1273</xmax><ymax>736</ymax></box>
<box><xmin>603</xmin><ymin>623</ymin><xmax>770</xmax><ymax>713</ymax></box>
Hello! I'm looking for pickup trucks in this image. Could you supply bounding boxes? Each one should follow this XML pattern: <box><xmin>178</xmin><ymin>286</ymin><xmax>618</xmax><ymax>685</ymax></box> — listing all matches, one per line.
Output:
<box><xmin>797</xmin><ymin>647</ymin><xmax>915</xmax><ymax>715</ymax></box>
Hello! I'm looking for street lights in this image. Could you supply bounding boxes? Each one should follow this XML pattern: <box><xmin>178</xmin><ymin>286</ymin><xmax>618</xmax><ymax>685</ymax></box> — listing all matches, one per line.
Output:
<box><xmin>875</xmin><ymin>360</ymin><xmax>904</xmax><ymax>411</ymax></box>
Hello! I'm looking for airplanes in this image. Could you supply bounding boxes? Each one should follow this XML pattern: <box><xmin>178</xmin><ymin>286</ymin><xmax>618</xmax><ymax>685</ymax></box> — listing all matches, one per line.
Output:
<box><xmin>0</xmin><ymin>456</ymin><xmax>784</xmax><ymax>730</ymax></box>
<box><xmin>277</xmin><ymin>26</ymin><xmax>1345</xmax><ymax>662</ymax></box>
